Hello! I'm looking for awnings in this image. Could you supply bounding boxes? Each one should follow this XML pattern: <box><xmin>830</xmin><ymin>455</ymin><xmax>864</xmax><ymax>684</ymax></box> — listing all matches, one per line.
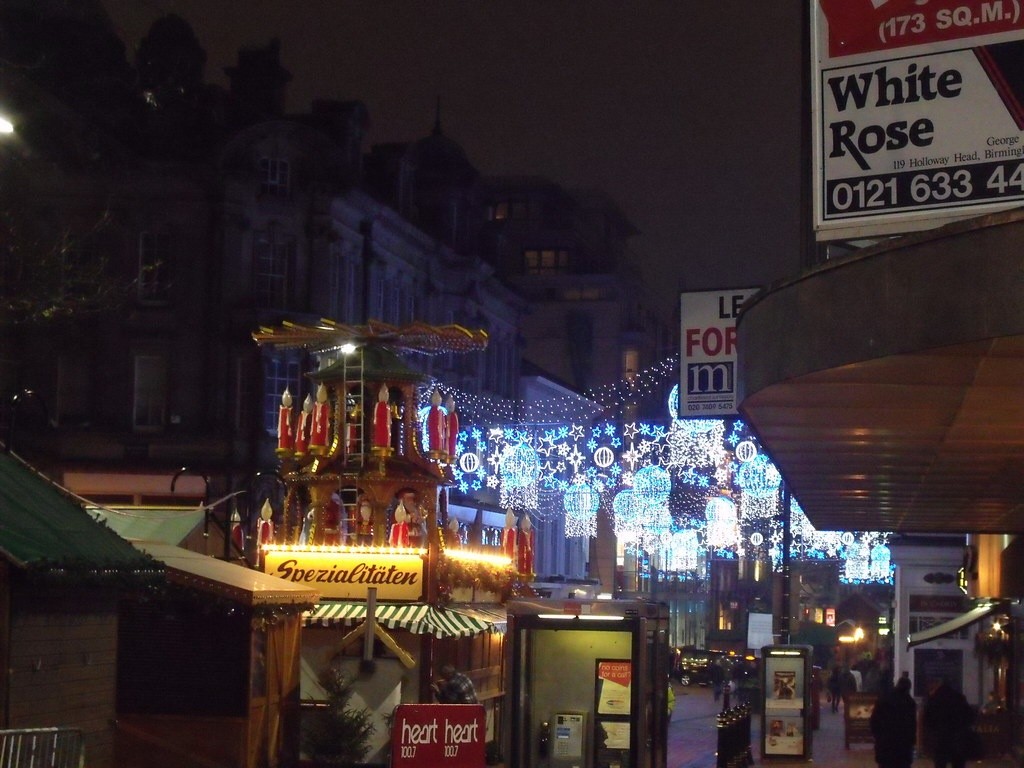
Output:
<box><xmin>299</xmin><ymin>602</ymin><xmax>507</xmax><ymax>639</ymax></box>
<box><xmin>906</xmin><ymin>602</ymin><xmax>995</xmax><ymax>650</ymax></box>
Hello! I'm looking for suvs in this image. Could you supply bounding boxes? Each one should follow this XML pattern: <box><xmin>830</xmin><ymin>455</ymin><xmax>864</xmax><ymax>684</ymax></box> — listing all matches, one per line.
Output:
<box><xmin>678</xmin><ymin>648</ymin><xmax>748</xmax><ymax>694</ymax></box>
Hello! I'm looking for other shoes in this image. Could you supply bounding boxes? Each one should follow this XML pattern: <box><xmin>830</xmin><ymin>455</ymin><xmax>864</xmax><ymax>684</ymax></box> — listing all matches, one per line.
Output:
<box><xmin>832</xmin><ymin>706</ymin><xmax>838</xmax><ymax>713</ymax></box>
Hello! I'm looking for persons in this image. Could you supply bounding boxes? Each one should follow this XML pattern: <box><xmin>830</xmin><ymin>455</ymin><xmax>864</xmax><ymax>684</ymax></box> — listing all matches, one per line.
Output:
<box><xmin>921</xmin><ymin>668</ymin><xmax>975</xmax><ymax>768</ymax></box>
<box><xmin>669</xmin><ymin>647</ymin><xmax>676</xmax><ymax>678</ymax></box>
<box><xmin>983</xmin><ymin>691</ymin><xmax>1001</xmax><ymax>710</ymax></box>
<box><xmin>429</xmin><ymin>661</ymin><xmax>480</xmax><ymax>704</ymax></box>
<box><xmin>828</xmin><ymin>661</ymin><xmax>857</xmax><ymax>714</ymax></box>
<box><xmin>870</xmin><ymin>677</ymin><xmax>917</xmax><ymax>768</ymax></box>
<box><xmin>667</xmin><ymin>684</ymin><xmax>676</xmax><ymax>723</ymax></box>
<box><xmin>711</xmin><ymin>659</ymin><xmax>723</xmax><ymax>703</ymax></box>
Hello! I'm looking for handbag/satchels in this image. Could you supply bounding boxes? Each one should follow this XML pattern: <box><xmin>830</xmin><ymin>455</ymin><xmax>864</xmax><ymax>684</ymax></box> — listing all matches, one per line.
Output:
<box><xmin>825</xmin><ymin>693</ymin><xmax>831</xmax><ymax>703</ymax></box>
